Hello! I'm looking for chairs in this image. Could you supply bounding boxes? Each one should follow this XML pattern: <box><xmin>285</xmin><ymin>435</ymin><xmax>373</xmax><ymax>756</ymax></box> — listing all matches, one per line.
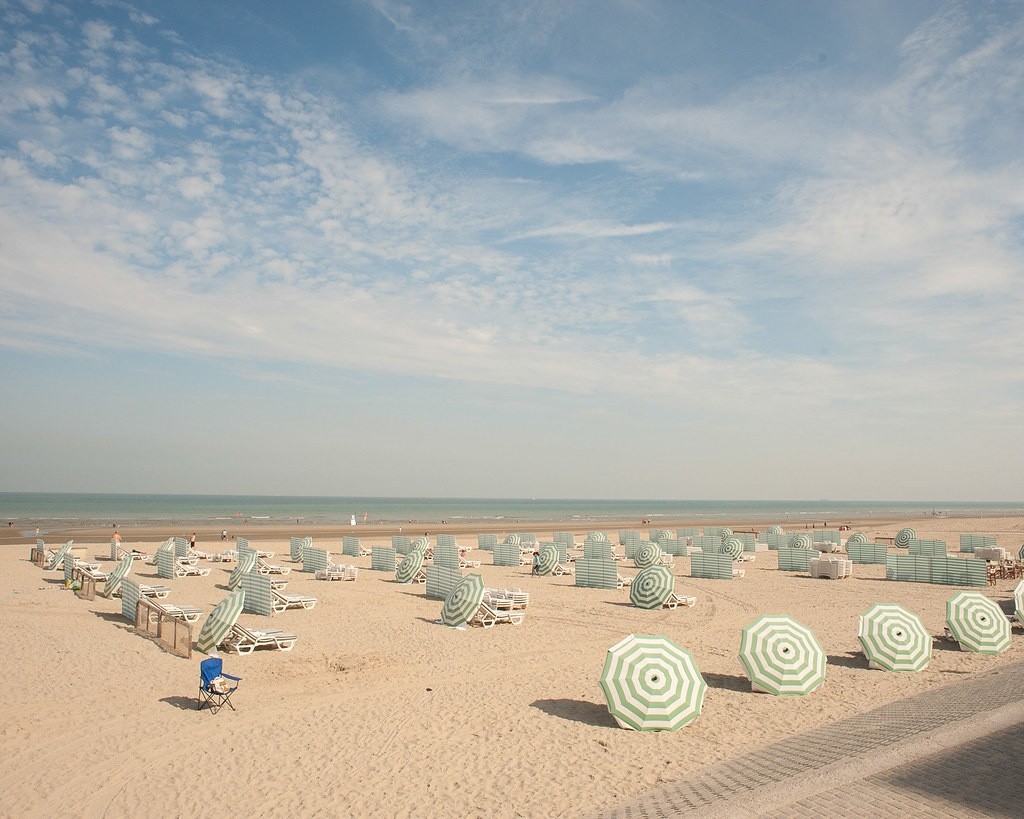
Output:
<box><xmin>468</xmin><ymin>588</ymin><xmax>530</xmax><ymax>628</ymax></box>
<box><xmin>731</xmin><ymin>568</ymin><xmax>747</xmax><ymax>579</ymax></box>
<box><xmin>72</xmin><ymin>556</ymin><xmax>111</xmax><ymax>583</ymax></box>
<box><xmin>741</xmin><ymin>554</ymin><xmax>756</xmax><ymax>563</ymax></box>
<box><xmin>617</xmin><ymin>573</ymin><xmax>635</xmax><ymax>587</ymax></box>
<box><xmin>259</xmin><ymin>560</ymin><xmax>292</xmax><ymax>575</ymax></box>
<box><xmin>810</xmin><ymin>538</ymin><xmax>854</xmax><ymax>581</ymax></box>
<box><xmin>566</xmin><ymin>552</ymin><xmax>580</xmax><ymax>563</ymax></box>
<box><xmin>175</xmin><ymin>548</ymin><xmax>238</xmax><ymax>577</ymax></box>
<box><xmin>272</xmin><ymin>580</ymin><xmax>290</xmax><ymax>589</ymax></box>
<box><xmin>139</xmin><ymin>582</ymin><xmax>173</xmax><ymax>598</ymax></box>
<box><xmin>259</xmin><ymin>551</ymin><xmax>275</xmax><ymax>559</ymax></box>
<box><xmin>416</xmin><ymin>569</ymin><xmax>426</xmax><ymax>585</ymax></box>
<box><xmin>575</xmin><ymin>542</ymin><xmax>583</xmax><ymax>549</ymax></box>
<box><xmin>197</xmin><ymin>658</ymin><xmax>243</xmax><ymax>715</ymax></box>
<box><xmin>117</xmin><ymin>546</ymin><xmax>151</xmax><ymax>561</ymax></box>
<box><xmin>457</xmin><ymin>544</ymin><xmax>481</xmax><ymax>570</ymax></box>
<box><xmin>223</xmin><ymin>623</ymin><xmax>298</xmax><ymax>656</ymax></box>
<box><xmin>554</xmin><ymin>565</ymin><xmax>574</xmax><ymax>577</ymax></box>
<box><xmin>360</xmin><ymin>543</ymin><xmax>372</xmax><ymax>556</ymax></box>
<box><xmin>273</xmin><ymin>590</ymin><xmax>318</xmax><ymax>613</ymax></box>
<box><xmin>46</xmin><ymin>547</ymin><xmax>81</xmax><ymax>563</ymax></box>
<box><xmin>144</xmin><ymin>603</ymin><xmax>203</xmax><ymax>625</ymax></box>
<box><xmin>610</xmin><ymin>542</ymin><xmax>627</xmax><ymax>562</ymax></box>
<box><xmin>670</xmin><ymin>593</ymin><xmax>696</xmax><ymax>610</ymax></box>
<box><xmin>517</xmin><ymin>540</ymin><xmax>538</xmax><ymax>555</ymax></box>
<box><xmin>426</xmin><ymin>547</ymin><xmax>434</xmax><ymax>560</ymax></box>
<box><xmin>976</xmin><ymin>545</ymin><xmax>1024</xmax><ymax>584</ymax></box>
<box><xmin>660</xmin><ymin>554</ymin><xmax>675</xmax><ymax>570</ymax></box>
<box><xmin>520</xmin><ymin>555</ymin><xmax>533</xmax><ymax>565</ymax></box>
<box><xmin>316</xmin><ymin>563</ymin><xmax>359</xmax><ymax>581</ymax></box>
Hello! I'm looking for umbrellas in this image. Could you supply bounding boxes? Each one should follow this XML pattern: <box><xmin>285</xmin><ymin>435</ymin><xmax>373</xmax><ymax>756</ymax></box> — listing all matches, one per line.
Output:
<box><xmin>440</xmin><ymin>572</ymin><xmax>485</xmax><ymax>627</ymax></box>
<box><xmin>599</xmin><ymin>632</ymin><xmax>708</xmax><ymax>733</ymax></box>
<box><xmin>503</xmin><ymin>525</ymin><xmax>917</xmax><ymax>609</ymax></box>
<box><xmin>738</xmin><ymin>614</ymin><xmax>828</xmax><ymax>696</ymax></box>
<box><xmin>944</xmin><ymin>590</ymin><xmax>1012</xmax><ymax>655</ymax></box>
<box><xmin>48</xmin><ymin>537</ymin><xmax>175</xmax><ymax>597</ymax></box>
<box><xmin>857</xmin><ymin>602</ymin><xmax>933</xmax><ymax>673</ymax></box>
<box><xmin>291</xmin><ymin>535</ymin><xmax>430</xmax><ymax>583</ymax></box>
<box><xmin>227</xmin><ymin>552</ymin><xmax>257</xmax><ymax>590</ymax></box>
<box><xmin>1013</xmin><ymin>545</ymin><xmax>1024</xmax><ymax>625</ymax></box>
<box><xmin>195</xmin><ymin>589</ymin><xmax>245</xmax><ymax>656</ymax></box>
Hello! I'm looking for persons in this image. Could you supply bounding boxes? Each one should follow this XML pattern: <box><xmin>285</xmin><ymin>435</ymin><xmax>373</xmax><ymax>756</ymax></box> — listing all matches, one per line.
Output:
<box><xmin>460</xmin><ymin>550</ymin><xmax>467</xmax><ymax>561</ymax></box>
<box><xmin>8</xmin><ymin>520</ymin><xmax>15</xmax><ymax>528</ymax></box>
<box><xmin>399</xmin><ymin>520</ymin><xmax>448</xmax><ymax>545</ymax></box>
<box><xmin>641</xmin><ymin>518</ymin><xmax>651</xmax><ymax>525</ymax></box>
<box><xmin>363</xmin><ymin>512</ymin><xmax>367</xmax><ymax>522</ymax></box>
<box><xmin>112</xmin><ymin>521</ymin><xmax>122</xmax><ymax>549</ymax></box>
<box><xmin>532</xmin><ymin>552</ymin><xmax>541</xmax><ymax>577</ymax></box>
<box><xmin>35</xmin><ymin>526</ymin><xmax>39</xmax><ymax>536</ymax></box>
<box><xmin>806</xmin><ymin>521</ymin><xmax>852</xmax><ymax>531</ymax></box>
<box><xmin>296</xmin><ymin>519</ymin><xmax>299</xmax><ymax>525</ymax></box>
<box><xmin>187</xmin><ymin>532</ymin><xmax>195</xmax><ymax>548</ymax></box>
<box><xmin>221</xmin><ymin>530</ymin><xmax>235</xmax><ymax>541</ymax></box>
